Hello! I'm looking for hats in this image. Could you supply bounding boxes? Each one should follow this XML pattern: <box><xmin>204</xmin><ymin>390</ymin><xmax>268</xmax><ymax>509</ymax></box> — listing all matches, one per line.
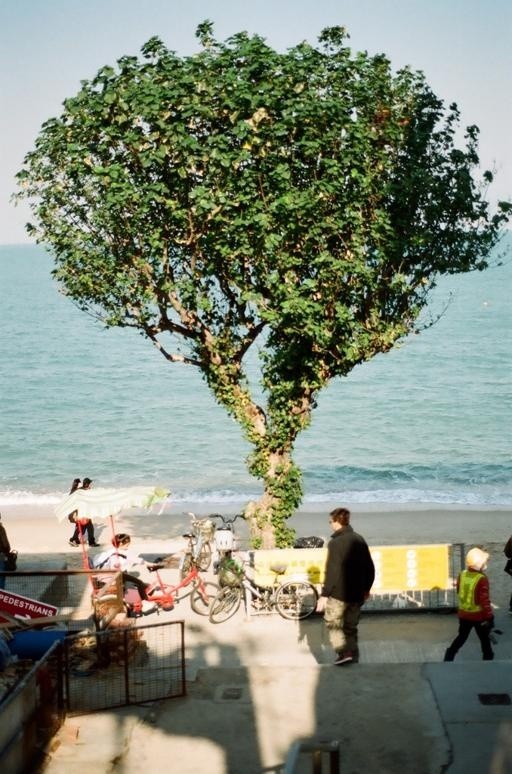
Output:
<box><xmin>466</xmin><ymin>547</ymin><xmax>490</xmax><ymax>572</ymax></box>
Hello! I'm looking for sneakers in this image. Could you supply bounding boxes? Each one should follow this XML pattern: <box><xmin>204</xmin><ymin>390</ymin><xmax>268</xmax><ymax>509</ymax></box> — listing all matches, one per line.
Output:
<box><xmin>89</xmin><ymin>542</ymin><xmax>100</xmax><ymax>547</ymax></box>
<box><xmin>333</xmin><ymin>652</ymin><xmax>354</xmax><ymax>666</ymax></box>
<box><xmin>69</xmin><ymin>538</ymin><xmax>80</xmax><ymax>547</ymax></box>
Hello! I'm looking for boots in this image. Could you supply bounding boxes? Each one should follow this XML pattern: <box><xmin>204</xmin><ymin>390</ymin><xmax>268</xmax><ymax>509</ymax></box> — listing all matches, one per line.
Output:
<box><xmin>442</xmin><ymin>648</ymin><xmax>457</xmax><ymax>662</ymax></box>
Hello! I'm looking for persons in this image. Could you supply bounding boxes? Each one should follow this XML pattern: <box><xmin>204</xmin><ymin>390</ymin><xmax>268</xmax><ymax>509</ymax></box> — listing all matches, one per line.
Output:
<box><xmin>445</xmin><ymin>548</ymin><xmax>494</xmax><ymax>661</ymax></box>
<box><xmin>315</xmin><ymin>508</ymin><xmax>374</xmax><ymax>665</ymax></box>
<box><xmin>93</xmin><ymin>534</ymin><xmax>164</xmax><ymax>600</ymax></box>
<box><xmin>504</xmin><ymin>534</ymin><xmax>512</xmax><ymax>612</ymax></box>
<box><xmin>68</xmin><ymin>478</ymin><xmax>99</xmax><ymax>547</ymax></box>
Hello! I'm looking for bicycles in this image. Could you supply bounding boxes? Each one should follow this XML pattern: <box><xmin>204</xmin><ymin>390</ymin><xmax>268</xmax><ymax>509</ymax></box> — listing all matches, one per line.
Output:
<box><xmin>78</xmin><ymin>512</ymin><xmax>319</xmax><ymax>624</ymax></box>
